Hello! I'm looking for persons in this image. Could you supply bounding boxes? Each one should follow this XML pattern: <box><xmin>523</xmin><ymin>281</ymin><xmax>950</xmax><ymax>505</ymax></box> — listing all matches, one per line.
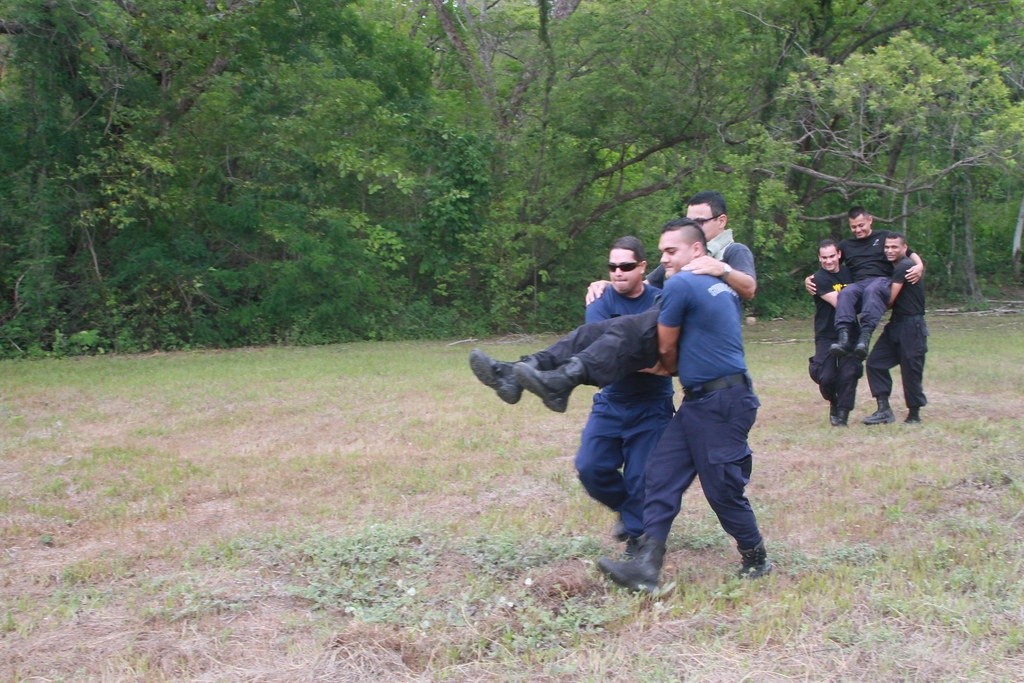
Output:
<box><xmin>598</xmin><ymin>217</ymin><xmax>770</xmax><ymax>595</ymax></box>
<box><xmin>469</xmin><ymin>194</ymin><xmax>759</xmax><ymax>413</ymax></box>
<box><xmin>805</xmin><ymin>206</ymin><xmax>924</xmax><ymax>358</ymax></box>
<box><xmin>863</xmin><ymin>233</ymin><xmax>927</xmax><ymax>426</ymax></box>
<box><xmin>808</xmin><ymin>239</ymin><xmax>864</xmax><ymax>426</ymax></box>
<box><xmin>572</xmin><ymin>236</ymin><xmax>672</xmax><ymax>549</ymax></box>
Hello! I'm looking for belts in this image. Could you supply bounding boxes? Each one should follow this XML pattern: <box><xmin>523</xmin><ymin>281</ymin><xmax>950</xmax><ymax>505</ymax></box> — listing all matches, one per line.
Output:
<box><xmin>891</xmin><ymin>314</ymin><xmax>923</xmax><ymax>323</ymax></box>
<box><xmin>684</xmin><ymin>373</ymin><xmax>746</xmax><ymax>400</ymax></box>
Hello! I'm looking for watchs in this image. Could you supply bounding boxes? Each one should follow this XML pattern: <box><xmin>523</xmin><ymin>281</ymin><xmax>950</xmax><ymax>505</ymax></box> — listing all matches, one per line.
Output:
<box><xmin>721</xmin><ymin>263</ymin><xmax>732</xmax><ymax>281</ymax></box>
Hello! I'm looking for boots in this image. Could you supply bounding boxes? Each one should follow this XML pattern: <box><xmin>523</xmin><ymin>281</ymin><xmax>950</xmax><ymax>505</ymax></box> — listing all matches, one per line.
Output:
<box><xmin>724</xmin><ymin>537</ymin><xmax>772</xmax><ymax>584</ymax></box>
<box><xmin>513</xmin><ymin>357</ymin><xmax>586</xmax><ymax>413</ymax></box>
<box><xmin>853</xmin><ymin>332</ymin><xmax>871</xmax><ymax>360</ymax></box>
<box><xmin>903</xmin><ymin>408</ymin><xmax>921</xmax><ymax>424</ymax></box>
<box><xmin>624</xmin><ymin>533</ymin><xmax>648</xmax><ymax>559</ymax></box>
<box><xmin>611</xmin><ymin>512</ymin><xmax>627</xmax><ymax>538</ymax></box>
<box><xmin>469</xmin><ymin>348</ymin><xmax>541</xmax><ymax>404</ymax></box>
<box><xmin>830</xmin><ymin>403</ymin><xmax>849</xmax><ymax>428</ymax></box>
<box><xmin>597</xmin><ymin>536</ymin><xmax>667</xmax><ymax>593</ymax></box>
<box><xmin>830</xmin><ymin>331</ymin><xmax>851</xmax><ymax>357</ymax></box>
<box><xmin>862</xmin><ymin>399</ymin><xmax>896</xmax><ymax>424</ymax></box>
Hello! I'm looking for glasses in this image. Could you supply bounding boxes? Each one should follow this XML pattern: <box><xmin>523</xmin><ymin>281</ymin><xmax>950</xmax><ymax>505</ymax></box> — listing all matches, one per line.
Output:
<box><xmin>693</xmin><ymin>213</ymin><xmax>722</xmax><ymax>226</ymax></box>
<box><xmin>608</xmin><ymin>262</ymin><xmax>640</xmax><ymax>272</ymax></box>
